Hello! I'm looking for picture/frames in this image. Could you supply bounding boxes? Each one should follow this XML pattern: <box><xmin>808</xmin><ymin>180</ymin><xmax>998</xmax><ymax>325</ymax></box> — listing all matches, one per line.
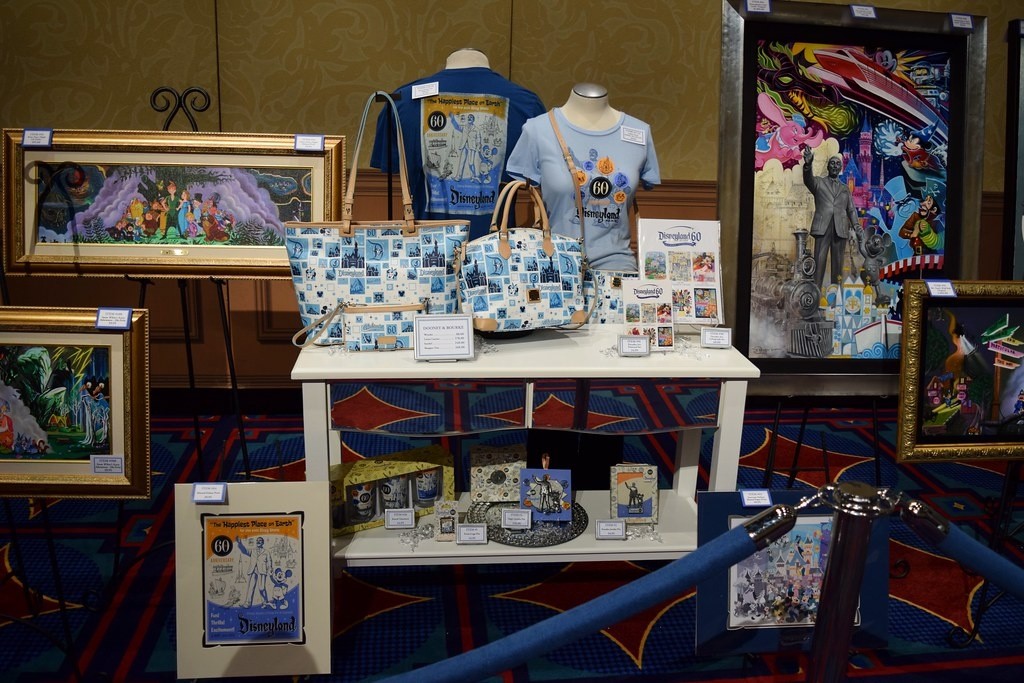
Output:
<box><xmin>0</xmin><ymin>306</ymin><xmax>151</xmax><ymax>501</ymax></box>
<box><xmin>695</xmin><ymin>491</ymin><xmax>891</xmax><ymax>657</ymax></box>
<box><xmin>894</xmin><ymin>279</ymin><xmax>1024</xmax><ymax>464</ymax></box>
<box><xmin>173</xmin><ymin>481</ymin><xmax>331</xmax><ymax>679</ymax></box>
<box><xmin>0</xmin><ymin>127</ymin><xmax>346</xmax><ymax>281</ymax></box>
<box><xmin>715</xmin><ymin>0</ymin><xmax>987</xmax><ymax>397</ymax></box>
<box><xmin>609</xmin><ymin>465</ymin><xmax>660</xmax><ymax>526</ymax></box>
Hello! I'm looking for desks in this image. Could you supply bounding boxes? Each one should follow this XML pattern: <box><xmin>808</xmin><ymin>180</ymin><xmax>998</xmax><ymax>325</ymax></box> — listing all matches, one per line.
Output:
<box><xmin>290</xmin><ymin>323</ymin><xmax>761</xmax><ymax>567</ymax></box>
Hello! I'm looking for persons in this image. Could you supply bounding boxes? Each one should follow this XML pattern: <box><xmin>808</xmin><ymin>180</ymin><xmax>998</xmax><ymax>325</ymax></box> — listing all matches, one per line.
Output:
<box><xmin>505</xmin><ymin>80</ymin><xmax>661</xmax><ymax>278</ymax></box>
<box><xmin>368</xmin><ymin>47</ymin><xmax>548</xmax><ymax>243</ymax></box>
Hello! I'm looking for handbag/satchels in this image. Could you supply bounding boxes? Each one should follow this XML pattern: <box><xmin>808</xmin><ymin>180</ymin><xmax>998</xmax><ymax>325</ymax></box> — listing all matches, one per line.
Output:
<box><xmin>451</xmin><ymin>181</ymin><xmax>586</xmax><ymax>334</ymax></box>
<box><xmin>285</xmin><ymin>92</ymin><xmax>469</xmax><ymax>347</ymax></box>
<box><xmin>582</xmin><ymin>267</ymin><xmax>639</xmax><ymax>325</ymax></box>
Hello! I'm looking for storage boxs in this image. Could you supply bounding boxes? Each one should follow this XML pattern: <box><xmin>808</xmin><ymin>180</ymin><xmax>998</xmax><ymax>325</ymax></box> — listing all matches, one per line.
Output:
<box><xmin>330</xmin><ymin>444</ymin><xmax>456</xmax><ymax>536</ymax></box>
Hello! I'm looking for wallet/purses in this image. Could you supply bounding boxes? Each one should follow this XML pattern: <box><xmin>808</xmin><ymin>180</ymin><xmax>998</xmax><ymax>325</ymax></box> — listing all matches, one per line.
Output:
<box><xmin>292</xmin><ymin>298</ymin><xmax>429</xmax><ymax>352</ymax></box>
